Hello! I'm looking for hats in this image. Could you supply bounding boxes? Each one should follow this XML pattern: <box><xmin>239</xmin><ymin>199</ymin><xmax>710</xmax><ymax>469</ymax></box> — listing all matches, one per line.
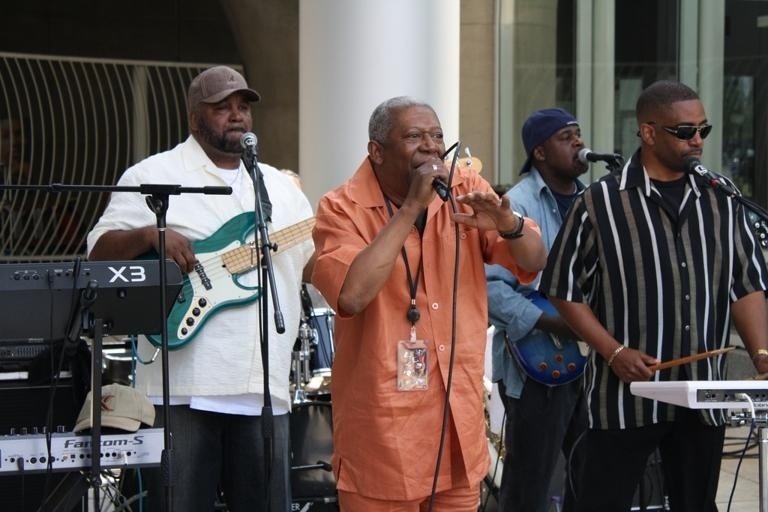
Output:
<box><xmin>519</xmin><ymin>108</ymin><xmax>580</xmax><ymax>177</ymax></box>
<box><xmin>187</xmin><ymin>64</ymin><xmax>261</xmax><ymax>113</ymax></box>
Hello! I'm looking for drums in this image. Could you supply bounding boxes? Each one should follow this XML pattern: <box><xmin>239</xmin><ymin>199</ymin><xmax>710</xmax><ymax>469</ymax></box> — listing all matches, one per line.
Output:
<box><xmin>288</xmin><ymin>306</ymin><xmax>335</xmax><ymax>394</ymax></box>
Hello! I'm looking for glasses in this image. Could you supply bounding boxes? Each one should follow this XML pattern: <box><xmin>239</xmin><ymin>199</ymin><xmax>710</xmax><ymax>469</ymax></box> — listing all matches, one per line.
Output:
<box><xmin>637</xmin><ymin>120</ymin><xmax>713</xmax><ymax>142</ymax></box>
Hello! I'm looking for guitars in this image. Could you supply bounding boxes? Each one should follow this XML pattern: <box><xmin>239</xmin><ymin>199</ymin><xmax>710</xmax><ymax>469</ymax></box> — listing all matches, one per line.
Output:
<box><xmin>505</xmin><ymin>287</ymin><xmax>590</xmax><ymax>387</ymax></box>
<box><xmin>133</xmin><ymin>147</ymin><xmax>483</xmax><ymax>351</ymax></box>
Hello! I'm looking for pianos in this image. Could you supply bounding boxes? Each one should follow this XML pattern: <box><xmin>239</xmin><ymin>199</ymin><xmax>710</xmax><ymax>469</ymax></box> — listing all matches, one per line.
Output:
<box><xmin>1</xmin><ymin>428</ymin><xmax>166</xmax><ymax>475</ymax></box>
<box><xmin>631</xmin><ymin>380</ymin><xmax>768</xmax><ymax>410</ymax></box>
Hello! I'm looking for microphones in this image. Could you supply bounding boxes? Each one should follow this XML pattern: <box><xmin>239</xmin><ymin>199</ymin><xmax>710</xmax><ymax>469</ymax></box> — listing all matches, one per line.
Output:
<box><xmin>431</xmin><ymin>176</ymin><xmax>448</xmax><ymax>202</ymax></box>
<box><xmin>578</xmin><ymin>148</ymin><xmax>621</xmax><ymax>164</ymax></box>
<box><xmin>240</xmin><ymin>132</ymin><xmax>258</xmax><ymax>159</ymax></box>
<box><xmin>682</xmin><ymin>156</ymin><xmax>714</xmax><ymax>186</ymax></box>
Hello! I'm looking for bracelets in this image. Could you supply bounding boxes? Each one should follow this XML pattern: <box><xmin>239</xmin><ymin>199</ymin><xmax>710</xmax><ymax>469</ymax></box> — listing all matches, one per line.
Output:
<box><xmin>750</xmin><ymin>349</ymin><xmax>767</xmax><ymax>360</ymax></box>
<box><xmin>608</xmin><ymin>345</ymin><xmax>624</xmax><ymax>367</ymax></box>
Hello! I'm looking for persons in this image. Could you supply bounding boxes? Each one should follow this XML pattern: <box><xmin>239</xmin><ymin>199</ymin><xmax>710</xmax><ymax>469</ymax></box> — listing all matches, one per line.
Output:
<box><xmin>85</xmin><ymin>65</ymin><xmax>318</xmax><ymax>512</ymax></box>
<box><xmin>310</xmin><ymin>96</ymin><xmax>550</xmax><ymax>511</ymax></box>
<box><xmin>538</xmin><ymin>81</ymin><xmax>767</xmax><ymax>512</ymax></box>
<box><xmin>483</xmin><ymin>107</ymin><xmax>590</xmax><ymax>512</ymax></box>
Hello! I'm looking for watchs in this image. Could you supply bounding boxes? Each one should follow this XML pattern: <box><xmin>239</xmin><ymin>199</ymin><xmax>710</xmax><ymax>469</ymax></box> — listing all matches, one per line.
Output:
<box><xmin>496</xmin><ymin>211</ymin><xmax>525</xmax><ymax>240</ymax></box>
